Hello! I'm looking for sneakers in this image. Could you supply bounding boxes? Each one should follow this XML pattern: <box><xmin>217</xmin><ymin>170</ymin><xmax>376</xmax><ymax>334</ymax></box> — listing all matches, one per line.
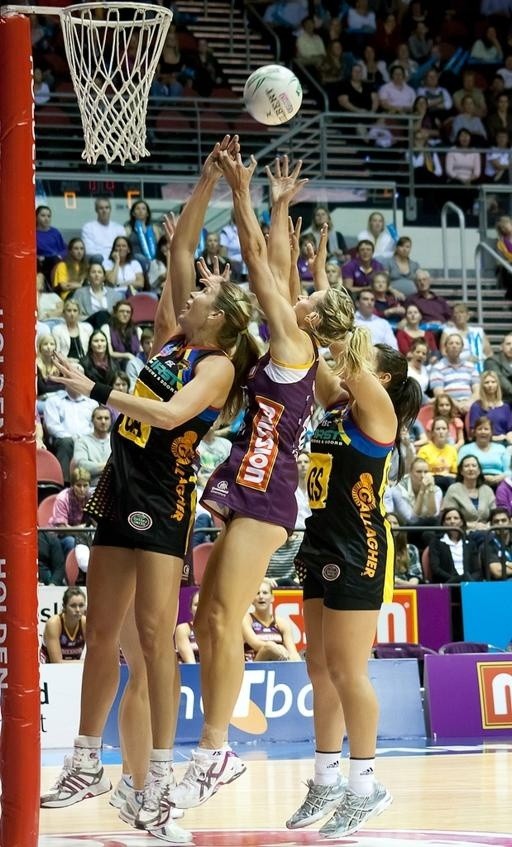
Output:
<box><xmin>318</xmin><ymin>781</ymin><xmax>392</xmax><ymax>839</ymax></box>
<box><xmin>40</xmin><ymin>751</ymin><xmax>113</xmax><ymax>810</ymax></box>
<box><xmin>109</xmin><ymin>778</ymin><xmax>133</xmax><ymax>810</ymax></box>
<box><xmin>166</xmin><ymin>746</ymin><xmax>247</xmax><ymax>809</ymax></box>
<box><xmin>118</xmin><ymin>787</ymin><xmax>193</xmax><ymax>844</ymax></box>
<box><xmin>285</xmin><ymin>774</ymin><xmax>348</xmax><ymax>830</ymax></box>
<box><xmin>135</xmin><ymin>768</ymin><xmax>177</xmax><ymax>831</ymax></box>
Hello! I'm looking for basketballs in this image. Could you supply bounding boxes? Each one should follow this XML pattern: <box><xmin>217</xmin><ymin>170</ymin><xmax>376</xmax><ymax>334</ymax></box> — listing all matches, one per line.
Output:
<box><xmin>243</xmin><ymin>65</ymin><xmax>302</xmax><ymax>127</ymax></box>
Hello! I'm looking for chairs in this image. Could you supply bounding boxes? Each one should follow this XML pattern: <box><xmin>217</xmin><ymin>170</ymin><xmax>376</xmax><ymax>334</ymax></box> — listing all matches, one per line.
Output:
<box><xmin>34</xmin><ymin>0</ymin><xmax>270</xmax><ymax>141</ymax></box>
